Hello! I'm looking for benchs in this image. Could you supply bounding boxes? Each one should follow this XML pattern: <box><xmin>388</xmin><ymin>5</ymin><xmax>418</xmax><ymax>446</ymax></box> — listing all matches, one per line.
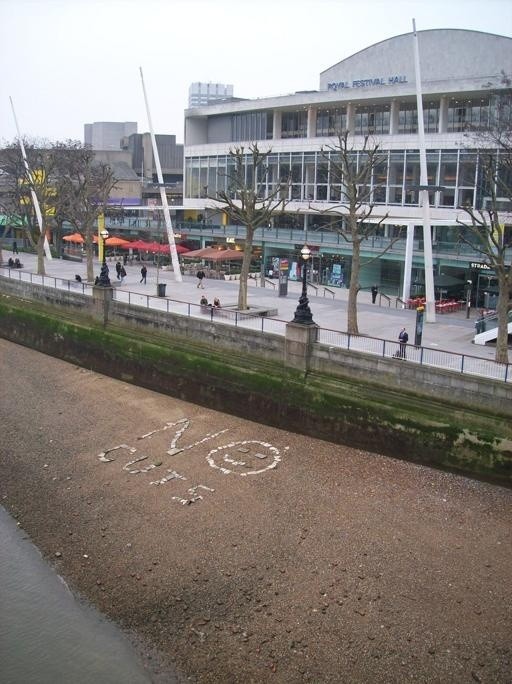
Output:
<box><xmin>63</xmin><ymin>278</ymin><xmax>121</xmax><ymax>288</ymax></box>
<box><xmin>208</xmin><ymin>303</ymin><xmax>278</xmax><ymax>321</ymax></box>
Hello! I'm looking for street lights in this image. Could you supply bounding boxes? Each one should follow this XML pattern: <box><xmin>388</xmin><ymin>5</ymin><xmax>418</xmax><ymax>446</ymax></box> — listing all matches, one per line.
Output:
<box><xmin>294</xmin><ymin>244</ymin><xmax>313</xmax><ymax>322</ymax></box>
<box><xmin>99</xmin><ymin>230</ymin><xmax>109</xmax><ymax>285</ymax></box>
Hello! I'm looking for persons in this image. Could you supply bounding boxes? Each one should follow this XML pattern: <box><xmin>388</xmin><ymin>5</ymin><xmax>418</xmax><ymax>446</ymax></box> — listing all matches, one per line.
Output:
<box><xmin>76</xmin><ymin>273</ymin><xmax>82</xmax><ymax>282</ymax></box>
<box><xmin>116</xmin><ymin>261</ymin><xmax>121</xmax><ymax>279</ymax></box>
<box><xmin>94</xmin><ymin>275</ymin><xmax>100</xmax><ymax>285</ymax></box>
<box><xmin>212</xmin><ymin>296</ymin><xmax>221</xmax><ymax>316</ymax></box>
<box><xmin>398</xmin><ymin>327</ymin><xmax>408</xmax><ymax>361</ymax></box>
<box><xmin>121</xmin><ymin>265</ymin><xmax>127</xmax><ymax>281</ymax></box>
<box><xmin>16</xmin><ymin>257</ymin><xmax>21</xmax><ymax>268</ymax></box>
<box><xmin>139</xmin><ymin>264</ymin><xmax>148</xmax><ymax>283</ymax></box>
<box><xmin>13</xmin><ymin>239</ymin><xmax>19</xmax><ymax>254</ymax></box>
<box><xmin>196</xmin><ymin>267</ymin><xmax>205</xmax><ymax>288</ymax></box>
<box><xmin>8</xmin><ymin>257</ymin><xmax>16</xmax><ymax>268</ymax></box>
<box><xmin>370</xmin><ymin>283</ymin><xmax>378</xmax><ymax>304</ymax></box>
<box><xmin>200</xmin><ymin>294</ymin><xmax>209</xmax><ymax>314</ymax></box>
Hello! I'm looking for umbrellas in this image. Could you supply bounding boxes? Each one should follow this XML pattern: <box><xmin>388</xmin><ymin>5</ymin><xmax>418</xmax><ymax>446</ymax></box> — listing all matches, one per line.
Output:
<box><xmin>62</xmin><ymin>232</ymin><xmax>130</xmax><ymax>260</ymax></box>
<box><xmin>121</xmin><ymin>239</ymin><xmax>151</xmax><ymax>262</ymax></box>
<box><xmin>410</xmin><ymin>271</ymin><xmax>469</xmax><ymax>311</ymax></box>
<box><xmin>160</xmin><ymin>243</ymin><xmax>192</xmax><ymax>254</ymax></box>
<box><xmin>144</xmin><ymin>240</ymin><xmax>165</xmax><ymax>263</ymax></box>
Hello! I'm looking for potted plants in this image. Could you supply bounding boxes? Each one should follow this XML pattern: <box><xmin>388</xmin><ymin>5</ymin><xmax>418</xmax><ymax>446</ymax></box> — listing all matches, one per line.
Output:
<box><xmin>224</xmin><ymin>268</ymin><xmax>262</xmax><ymax>280</ymax></box>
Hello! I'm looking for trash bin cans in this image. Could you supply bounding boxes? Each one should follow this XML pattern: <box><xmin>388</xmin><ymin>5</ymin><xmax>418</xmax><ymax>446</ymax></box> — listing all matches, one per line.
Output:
<box><xmin>61</xmin><ymin>247</ymin><xmax>64</xmax><ymax>252</ymax></box>
<box><xmin>159</xmin><ymin>284</ymin><xmax>166</xmax><ymax>297</ymax></box>
<box><xmin>281</xmin><ymin>283</ymin><xmax>286</xmax><ymax>294</ymax></box>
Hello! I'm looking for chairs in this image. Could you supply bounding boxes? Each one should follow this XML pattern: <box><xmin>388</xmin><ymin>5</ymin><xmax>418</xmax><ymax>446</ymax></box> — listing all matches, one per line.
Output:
<box><xmin>409</xmin><ymin>297</ymin><xmax>461</xmax><ymax>313</ymax></box>
